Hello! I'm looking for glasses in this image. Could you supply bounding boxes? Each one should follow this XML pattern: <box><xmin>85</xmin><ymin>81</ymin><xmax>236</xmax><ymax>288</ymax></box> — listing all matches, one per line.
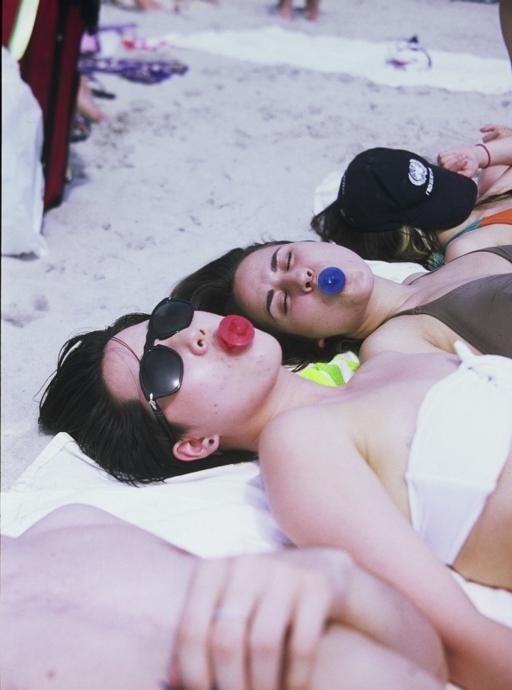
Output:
<box><xmin>137</xmin><ymin>297</ymin><xmax>194</xmax><ymax>443</ymax></box>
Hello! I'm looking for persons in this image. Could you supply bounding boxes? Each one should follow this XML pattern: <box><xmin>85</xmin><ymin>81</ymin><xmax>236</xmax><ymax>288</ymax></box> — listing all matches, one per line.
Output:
<box><xmin>167</xmin><ymin>239</ymin><xmax>512</xmax><ymax>364</ymax></box>
<box><xmin>480</xmin><ymin>124</ymin><xmax>512</xmax><ymax>142</ymax></box>
<box><xmin>34</xmin><ymin>297</ymin><xmax>512</xmax><ymax>690</ymax></box>
<box><xmin>309</xmin><ymin>136</ymin><xmax>512</xmax><ymax>264</ymax></box>
<box><xmin>278</xmin><ymin>0</ymin><xmax>320</xmax><ymax>18</ymax></box>
<box><xmin>0</xmin><ymin>501</ymin><xmax>450</xmax><ymax>690</ymax></box>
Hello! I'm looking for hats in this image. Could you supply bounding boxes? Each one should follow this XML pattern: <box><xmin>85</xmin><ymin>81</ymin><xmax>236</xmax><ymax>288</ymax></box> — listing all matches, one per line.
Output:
<box><xmin>338</xmin><ymin>148</ymin><xmax>478</xmax><ymax>234</ymax></box>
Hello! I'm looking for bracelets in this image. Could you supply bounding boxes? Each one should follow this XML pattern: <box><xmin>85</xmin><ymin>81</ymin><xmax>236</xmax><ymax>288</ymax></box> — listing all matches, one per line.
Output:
<box><xmin>475</xmin><ymin>144</ymin><xmax>491</xmax><ymax>168</ymax></box>
